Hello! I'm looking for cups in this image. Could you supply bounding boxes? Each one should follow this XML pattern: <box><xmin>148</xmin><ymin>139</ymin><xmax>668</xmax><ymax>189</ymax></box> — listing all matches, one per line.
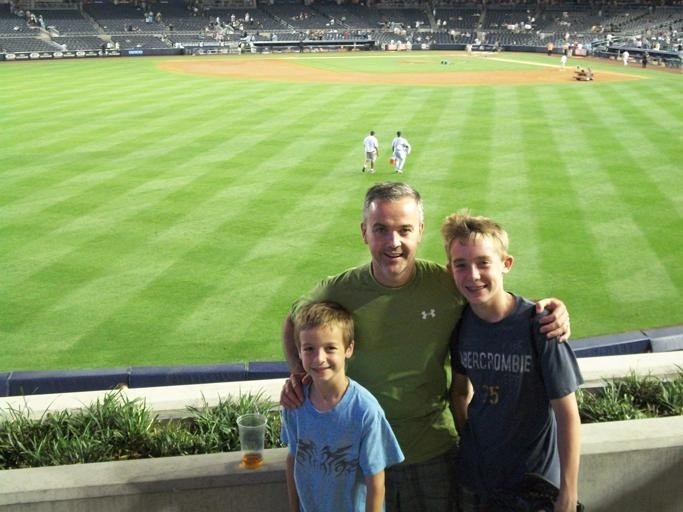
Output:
<box><xmin>235</xmin><ymin>413</ymin><xmax>268</xmax><ymax>468</ymax></box>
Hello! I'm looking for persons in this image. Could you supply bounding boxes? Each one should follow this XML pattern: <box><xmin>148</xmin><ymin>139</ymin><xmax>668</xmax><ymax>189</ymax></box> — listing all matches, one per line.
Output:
<box><xmin>392</xmin><ymin>131</ymin><xmax>411</xmax><ymax>173</ymax></box>
<box><xmin>362</xmin><ymin>131</ymin><xmax>380</xmax><ymax>174</ymax></box>
<box><xmin>441</xmin><ymin>210</ymin><xmax>584</xmax><ymax>512</ymax></box>
<box><xmin>9</xmin><ymin>1</ymin><xmax>683</xmax><ymax>81</ymax></box>
<box><xmin>280</xmin><ymin>300</ymin><xmax>405</xmax><ymax>512</ymax></box>
<box><xmin>280</xmin><ymin>183</ymin><xmax>570</xmax><ymax>512</ymax></box>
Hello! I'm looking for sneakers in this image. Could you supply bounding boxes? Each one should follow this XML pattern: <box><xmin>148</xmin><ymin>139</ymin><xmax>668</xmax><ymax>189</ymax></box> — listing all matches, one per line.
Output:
<box><xmin>362</xmin><ymin>166</ymin><xmax>402</xmax><ymax>173</ymax></box>
